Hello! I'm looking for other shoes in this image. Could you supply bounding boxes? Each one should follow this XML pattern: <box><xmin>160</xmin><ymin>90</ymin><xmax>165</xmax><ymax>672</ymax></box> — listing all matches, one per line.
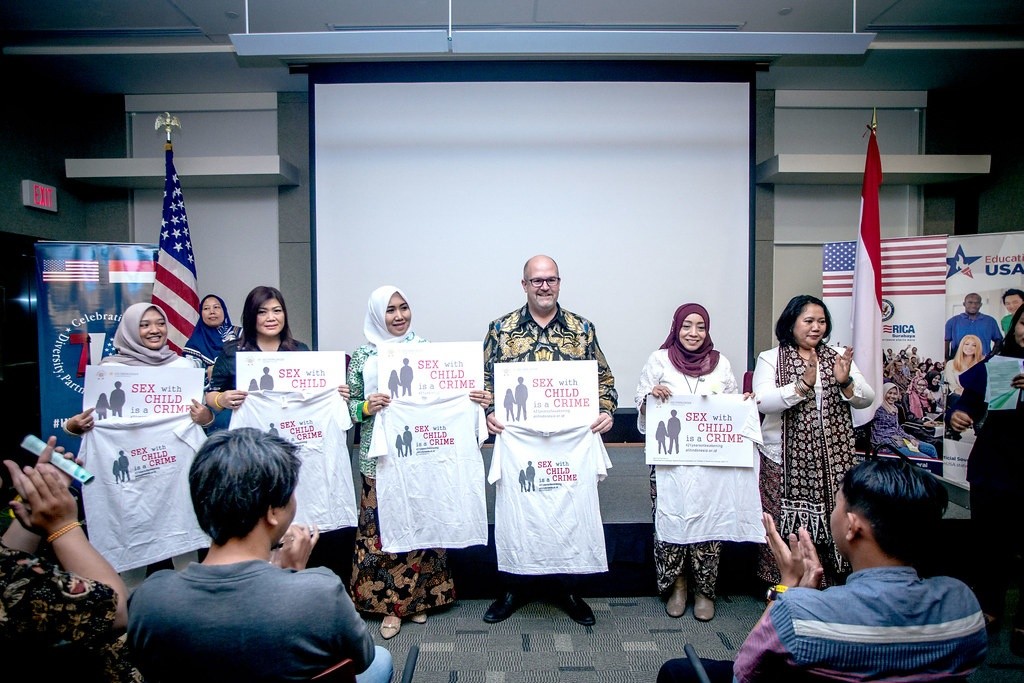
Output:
<box><xmin>983</xmin><ymin>614</ymin><xmax>1001</xmax><ymax>635</ymax></box>
<box><xmin>1010</xmin><ymin>627</ymin><xmax>1024</xmax><ymax>657</ymax></box>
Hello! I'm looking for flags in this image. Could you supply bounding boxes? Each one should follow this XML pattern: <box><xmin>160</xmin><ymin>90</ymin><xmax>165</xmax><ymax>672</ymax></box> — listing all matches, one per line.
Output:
<box><xmin>850</xmin><ymin>130</ymin><xmax>883</xmax><ymax>427</ymax></box>
<box><xmin>151</xmin><ymin>140</ymin><xmax>201</xmax><ymax>357</ymax></box>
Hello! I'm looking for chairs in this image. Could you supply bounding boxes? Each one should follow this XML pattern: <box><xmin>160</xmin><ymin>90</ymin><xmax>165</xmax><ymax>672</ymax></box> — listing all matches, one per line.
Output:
<box><xmin>309</xmin><ymin>644</ymin><xmax>420</xmax><ymax>683</ymax></box>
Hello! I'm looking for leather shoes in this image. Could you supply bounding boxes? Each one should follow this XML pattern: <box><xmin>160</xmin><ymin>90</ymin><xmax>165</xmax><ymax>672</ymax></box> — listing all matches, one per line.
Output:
<box><xmin>410</xmin><ymin>610</ymin><xmax>427</xmax><ymax>622</ymax></box>
<box><xmin>380</xmin><ymin>616</ymin><xmax>401</xmax><ymax>638</ymax></box>
<box><xmin>484</xmin><ymin>592</ymin><xmax>520</xmax><ymax>622</ymax></box>
<box><xmin>555</xmin><ymin>592</ymin><xmax>595</xmax><ymax>626</ymax></box>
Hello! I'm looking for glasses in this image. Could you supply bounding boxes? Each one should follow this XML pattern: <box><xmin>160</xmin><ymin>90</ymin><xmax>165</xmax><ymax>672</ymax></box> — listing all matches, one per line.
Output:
<box><xmin>917</xmin><ymin>384</ymin><xmax>927</xmax><ymax>387</ymax></box>
<box><xmin>524</xmin><ymin>277</ymin><xmax>560</xmax><ymax>287</ymax></box>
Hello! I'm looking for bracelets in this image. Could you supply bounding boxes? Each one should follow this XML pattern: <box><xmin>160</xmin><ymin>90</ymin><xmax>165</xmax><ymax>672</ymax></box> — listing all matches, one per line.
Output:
<box><xmin>215</xmin><ymin>393</ymin><xmax>222</xmax><ymax>408</ymax></box>
<box><xmin>364</xmin><ymin>401</ymin><xmax>371</xmax><ymax>416</ymax></box>
<box><xmin>63</xmin><ymin>421</ymin><xmax>79</xmax><ymax>436</ymax></box>
<box><xmin>840</xmin><ymin>376</ymin><xmax>853</xmax><ymax>388</ymax></box>
<box><xmin>202</xmin><ymin>412</ymin><xmax>215</xmax><ymax>426</ymax></box>
<box><xmin>47</xmin><ymin>522</ymin><xmax>82</xmax><ymax>542</ymax></box>
<box><xmin>795</xmin><ymin>378</ymin><xmax>815</xmax><ymax>397</ymax></box>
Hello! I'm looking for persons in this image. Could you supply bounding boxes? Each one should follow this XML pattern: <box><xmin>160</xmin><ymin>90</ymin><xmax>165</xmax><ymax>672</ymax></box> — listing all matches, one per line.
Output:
<box><xmin>206</xmin><ymin>286</ymin><xmax>351</xmax><ymax>577</ymax></box>
<box><xmin>869</xmin><ymin>289</ymin><xmax>1024</xmax><ymax>460</ymax></box>
<box><xmin>181</xmin><ymin>294</ymin><xmax>244</xmax><ymax>437</ymax></box>
<box><xmin>126</xmin><ymin>427</ymin><xmax>394</xmax><ymax>683</ymax></box>
<box><xmin>0</xmin><ymin>435</ymin><xmax>144</xmax><ymax>683</ymax></box>
<box><xmin>635</xmin><ymin>303</ymin><xmax>761</xmax><ymax>621</ymax></box>
<box><xmin>945</xmin><ymin>303</ymin><xmax>1024</xmax><ymax>658</ymax></box>
<box><xmin>733</xmin><ymin>458</ymin><xmax>988</xmax><ymax>683</ymax></box>
<box><xmin>63</xmin><ymin>302</ymin><xmax>214</xmax><ymax>579</ymax></box>
<box><xmin>752</xmin><ymin>295</ymin><xmax>875</xmax><ymax>606</ymax></box>
<box><xmin>483</xmin><ymin>255</ymin><xmax>618</xmax><ymax>626</ymax></box>
<box><xmin>347</xmin><ymin>286</ymin><xmax>491</xmax><ymax>640</ymax></box>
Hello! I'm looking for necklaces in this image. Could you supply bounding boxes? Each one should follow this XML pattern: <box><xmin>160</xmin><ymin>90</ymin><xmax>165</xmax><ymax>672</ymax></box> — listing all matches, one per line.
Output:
<box><xmin>797</xmin><ymin>351</ymin><xmax>810</xmax><ymax>361</ymax></box>
<box><xmin>683</xmin><ymin>374</ymin><xmax>700</xmax><ymax>395</ymax></box>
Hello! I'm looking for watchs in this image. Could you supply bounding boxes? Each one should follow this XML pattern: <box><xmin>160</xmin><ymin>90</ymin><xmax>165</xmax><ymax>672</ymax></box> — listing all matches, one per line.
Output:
<box><xmin>767</xmin><ymin>585</ymin><xmax>788</xmax><ymax>601</ymax></box>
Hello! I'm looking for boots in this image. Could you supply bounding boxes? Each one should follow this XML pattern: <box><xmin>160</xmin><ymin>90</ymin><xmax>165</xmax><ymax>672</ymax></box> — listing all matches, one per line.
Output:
<box><xmin>666</xmin><ymin>573</ymin><xmax>687</xmax><ymax>616</ymax></box>
<box><xmin>694</xmin><ymin>592</ymin><xmax>714</xmax><ymax>620</ymax></box>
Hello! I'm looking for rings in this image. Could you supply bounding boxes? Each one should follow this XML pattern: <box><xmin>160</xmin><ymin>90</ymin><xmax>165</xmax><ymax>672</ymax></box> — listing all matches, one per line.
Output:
<box><xmin>87</xmin><ymin>424</ymin><xmax>90</xmax><ymax>428</ymax></box>
<box><xmin>483</xmin><ymin>394</ymin><xmax>485</xmax><ymax>399</ymax></box>
<box><xmin>810</xmin><ymin>363</ymin><xmax>814</xmax><ymax>367</ymax></box>
<box><xmin>232</xmin><ymin>402</ymin><xmax>235</xmax><ymax>406</ymax></box>
